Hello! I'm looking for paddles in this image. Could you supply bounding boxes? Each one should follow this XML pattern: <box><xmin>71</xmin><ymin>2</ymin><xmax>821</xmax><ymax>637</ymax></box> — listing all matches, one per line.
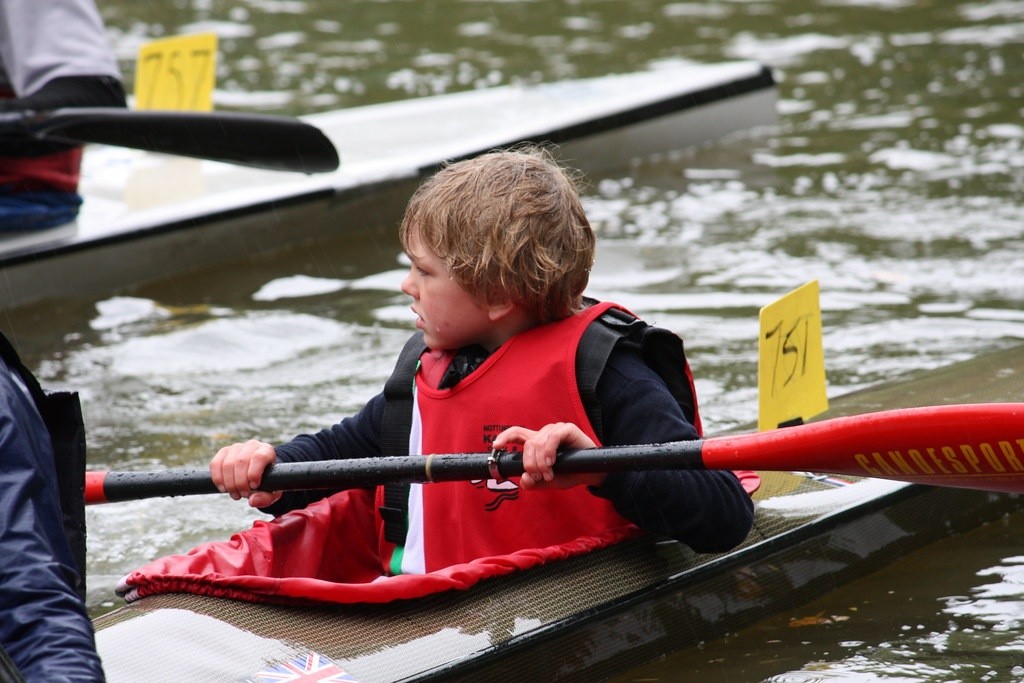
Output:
<box><xmin>80</xmin><ymin>395</ymin><xmax>1024</xmax><ymax>512</ymax></box>
<box><xmin>1</xmin><ymin>101</ymin><xmax>342</xmax><ymax>178</ymax></box>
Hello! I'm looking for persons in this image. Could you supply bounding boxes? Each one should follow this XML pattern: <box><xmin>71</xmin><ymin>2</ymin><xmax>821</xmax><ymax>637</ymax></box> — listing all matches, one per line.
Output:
<box><xmin>0</xmin><ymin>333</ymin><xmax>107</xmax><ymax>683</ymax></box>
<box><xmin>213</xmin><ymin>148</ymin><xmax>753</xmax><ymax>584</ymax></box>
<box><xmin>0</xmin><ymin>0</ymin><xmax>127</xmax><ymax>231</ymax></box>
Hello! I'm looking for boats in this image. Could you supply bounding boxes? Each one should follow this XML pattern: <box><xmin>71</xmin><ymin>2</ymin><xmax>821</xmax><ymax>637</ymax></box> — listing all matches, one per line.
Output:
<box><xmin>0</xmin><ymin>59</ymin><xmax>784</xmax><ymax>300</ymax></box>
<box><xmin>94</xmin><ymin>339</ymin><xmax>1024</xmax><ymax>683</ymax></box>
